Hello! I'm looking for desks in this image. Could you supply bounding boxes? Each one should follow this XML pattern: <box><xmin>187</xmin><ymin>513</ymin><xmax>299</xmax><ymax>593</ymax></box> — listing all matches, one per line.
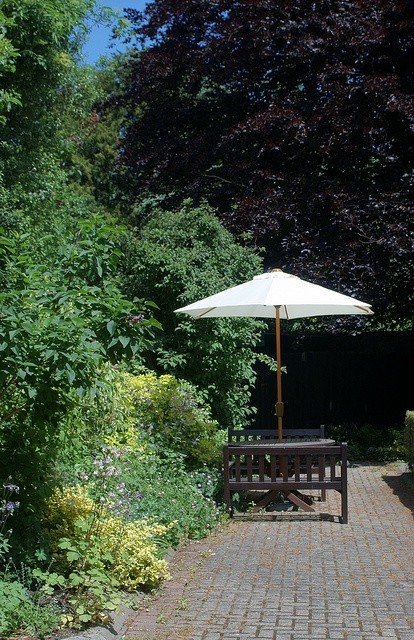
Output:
<box><xmin>224</xmin><ymin>438</ymin><xmax>341</xmax><ymax>513</ymax></box>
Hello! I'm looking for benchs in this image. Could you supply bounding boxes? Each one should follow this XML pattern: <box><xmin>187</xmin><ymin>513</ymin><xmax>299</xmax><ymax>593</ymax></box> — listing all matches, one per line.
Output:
<box><xmin>224</xmin><ymin>425</ymin><xmax>327</xmax><ymax>501</ymax></box>
<box><xmin>224</xmin><ymin>441</ymin><xmax>348</xmax><ymax>523</ymax></box>
